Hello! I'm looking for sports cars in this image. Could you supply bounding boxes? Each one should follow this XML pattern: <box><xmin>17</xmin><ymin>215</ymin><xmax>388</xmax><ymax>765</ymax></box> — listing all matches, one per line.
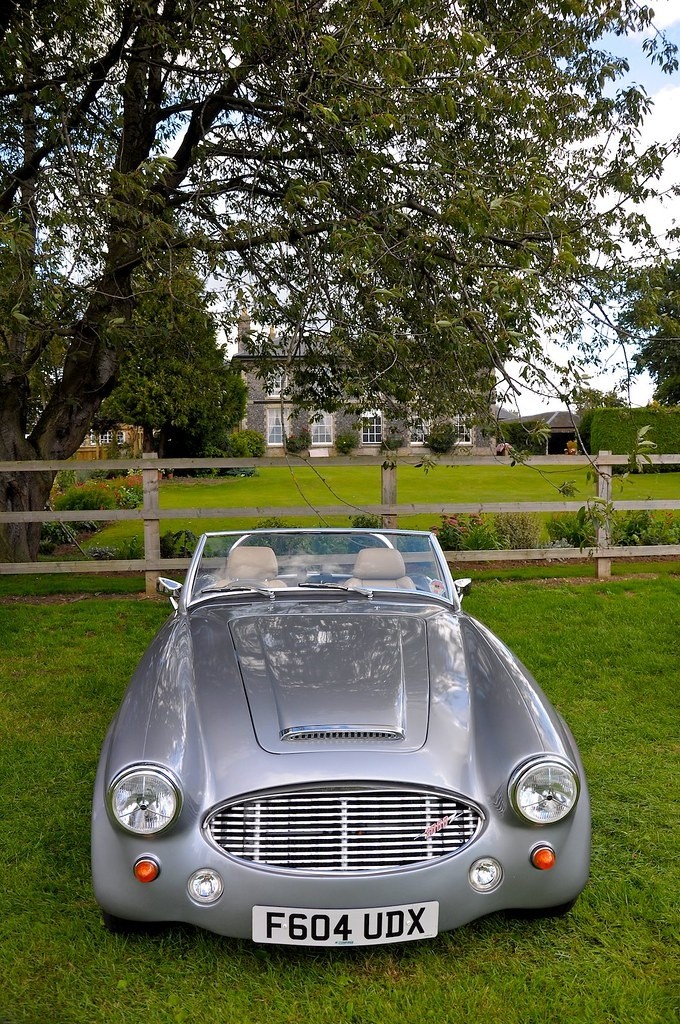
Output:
<box><xmin>89</xmin><ymin>526</ymin><xmax>592</xmax><ymax>955</ymax></box>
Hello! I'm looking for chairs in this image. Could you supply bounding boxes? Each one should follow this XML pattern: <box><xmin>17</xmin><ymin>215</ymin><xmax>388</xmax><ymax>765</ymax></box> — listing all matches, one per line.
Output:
<box><xmin>344</xmin><ymin>547</ymin><xmax>417</xmax><ymax>590</ymax></box>
<box><xmin>214</xmin><ymin>546</ymin><xmax>286</xmax><ymax>588</ymax></box>
